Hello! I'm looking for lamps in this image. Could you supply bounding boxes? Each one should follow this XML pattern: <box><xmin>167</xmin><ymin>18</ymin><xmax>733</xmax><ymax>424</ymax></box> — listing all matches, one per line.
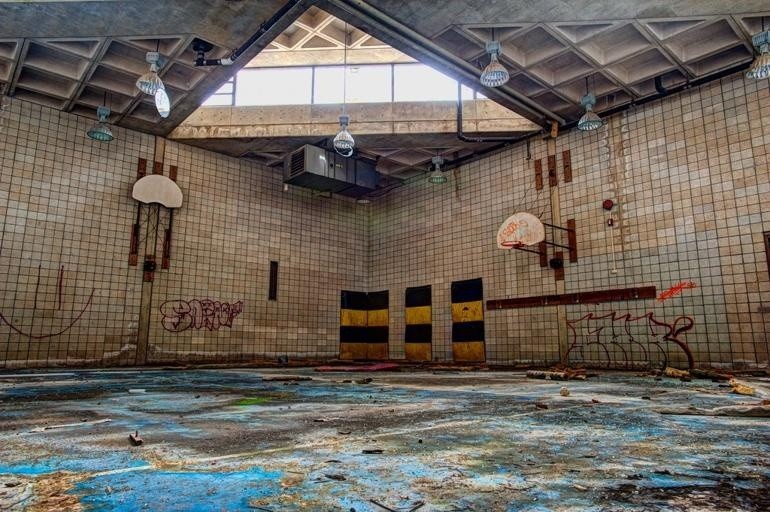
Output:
<box><xmin>576</xmin><ymin>78</ymin><xmax>603</xmax><ymax>130</ymax></box>
<box><xmin>87</xmin><ymin>91</ymin><xmax>114</xmax><ymax>141</ymax></box>
<box><xmin>480</xmin><ymin>28</ymin><xmax>509</xmax><ymax>87</ymax></box>
<box><xmin>428</xmin><ymin>148</ymin><xmax>447</xmax><ymax>184</ymax></box>
<box><xmin>333</xmin><ymin>22</ymin><xmax>355</xmax><ymax>148</ymax></box>
<box><xmin>745</xmin><ymin>16</ymin><xmax>770</xmax><ymax>80</ymax></box>
<box><xmin>135</xmin><ymin>39</ymin><xmax>165</xmax><ymax>96</ymax></box>
